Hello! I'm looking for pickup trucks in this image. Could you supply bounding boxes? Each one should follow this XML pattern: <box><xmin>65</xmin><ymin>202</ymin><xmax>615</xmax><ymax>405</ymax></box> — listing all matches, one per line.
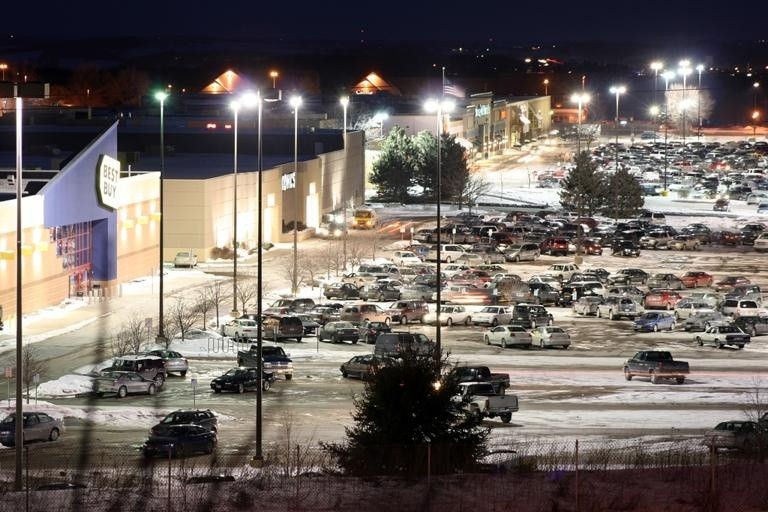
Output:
<box><xmin>721</xmin><ymin>300</ymin><xmax>767</xmax><ymax>319</ymax></box>
<box><xmin>440</xmin><ymin>365</ymin><xmax>511</xmax><ymax>394</ymax></box>
<box><xmin>235</xmin><ymin>344</ymin><xmax>294</xmax><ymax>382</ymax></box>
<box><xmin>449</xmin><ymin>380</ymin><xmax>519</xmax><ymax>424</ymax></box>
<box><xmin>684</xmin><ymin>311</ymin><xmax>730</xmax><ymax>330</ymax></box>
<box><xmin>623</xmin><ymin>349</ymin><xmax>690</xmax><ymax>384</ymax></box>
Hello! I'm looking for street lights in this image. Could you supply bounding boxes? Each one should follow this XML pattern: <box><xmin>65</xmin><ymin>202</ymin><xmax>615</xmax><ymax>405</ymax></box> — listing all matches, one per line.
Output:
<box><xmin>752</xmin><ymin>80</ymin><xmax>760</xmax><ymax>138</ymax></box>
<box><xmin>572</xmin><ymin>93</ymin><xmax>591</xmax><ymax>157</ymax></box>
<box><xmin>245</xmin><ymin>87</ymin><xmax>268</xmax><ymax>468</ymax></box>
<box><xmin>270</xmin><ymin>72</ymin><xmax>279</xmax><ymax>88</ymax></box>
<box><xmin>0</xmin><ymin>64</ymin><xmax>7</xmax><ymax>80</ymax></box>
<box><xmin>609</xmin><ymin>87</ymin><xmax>626</xmax><ymax>175</ymax></box>
<box><xmin>288</xmin><ymin>95</ymin><xmax>298</xmax><ymax>297</ymax></box>
<box><xmin>375</xmin><ymin>113</ymin><xmax>388</xmax><ymax>142</ymax></box>
<box><xmin>581</xmin><ymin>74</ymin><xmax>586</xmax><ymax>93</ymax></box>
<box><xmin>152</xmin><ymin>90</ymin><xmax>171</xmax><ymax>344</ymax></box>
<box><xmin>543</xmin><ymin>79</ymin><xmax>548</xmax><ymax>97</ymax></box>
<box><xmin>229</xmin><ymin>99</ymin><xmax>242</xmax><ymax>314</ymax></box>
<box><xmin>424</xmin><ymin>434</ymin><xmax>434</xmax><ymax>486</ymax></box>
<box><xmin>649</xmin><ymin>59</ymin><xmax>702</xmax><ymax>191</ymax></box>
<box><xmin>422</xmin><ymin>96</ymin><xmax>457</xmax><ymax>369</ymax></box>
<box><xmin>340</xmin><ymin>95</ymin><xmax>350</xmax><ymax>221</ymax></box>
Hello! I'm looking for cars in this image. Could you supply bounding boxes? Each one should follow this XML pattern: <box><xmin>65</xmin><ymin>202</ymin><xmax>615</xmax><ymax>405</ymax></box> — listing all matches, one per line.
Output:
<box><xmin>749</xmin><ymin>139</ymin><xmax>756</xmax><ymax>144</ymax></box>
<box><xmin>756</xmin><ymin>412</ymin><xmax>768</xmax><ymax>431</ymax></box>
<box><xmin>264</xmin><ymin>306</ymin><xmax>297</xmax><ymax>316</ymax></box>
<box><xmin>693</xmin><ymin>325</ymin><xmax>751</xmax><ymax>349</ymax></box>
<box><xmin>471</xmin><ymin>306</ymin><xmax>513</xmax><ymax>327</ymax></box>
<box><xmin>174</xmin><ymin>252</ymin><xmax>198</xmax><ymax>268</ymax></box>
<box><xmin>303</xmin><ymin>307</ymin><xmax>340</xmax><ymax>323</ymax></box>
<box><xmin>339</xmin><ymin>354</ymin><xmax>394</xmax><ymax>381</ymax></box>
<box><xmin>571</xmin><ymin>296</ymin><xmax>603</xmax><ymax>316</ymax></box>
<box><xmin>537</xmin><ymin>141</ymin><xmax>768</xmax><ymax>204</ymax></box>
<box><xmin>0</xmin><ymin>412</ymin><xmax>65</xmax><ymax>447</ymax></box>
<box><xmin>529</xmin><ymin>326</ymin><xmax>571</xmax><ymax>350</ymax></box>
<box><xmin>483</xmin><ymin>325</ymin><xmax>532</xmax><ymax>348</ymax></box>
<box><xmin>297</xmin><ymin>314</ymin><xmax>320</xmax><ymax>336</ymax></box>
<box><xmin>240</xmin><ymin>314</ymin><xmax>265</xmax><ymax>324</ymax></box>
<box><xmin>713</xmin><ymin>199</ymin><xmax>729</xmax><ymax>211</ymax></box>
<box><xmin>211</xmin><ymin>366</ymin><xmax>277</xmax><ymax>394</ymax></box>
<box><xmin>632</xmin><ymin>311</ymin><xmax>676</xmax><ymax>332</ymax></box>
<box><xmin>674</xmin><ymin>302</ymin><xmax>716</xmax><ymax>319</ymax></box>
<box><xmin>319</xmin><ymin>321</ymin><xmax>360</xmax><ymax>344</ymax></box>
<box><xmin>326</xmin><ymin>223</ymin><xmax>348</xmax><ymax>239</ymax></box>
<box><xmin>733</xmin><ymin>317</ymin><xmax>768</xmax><ymax>336</ymax></box>
<box><xmin>92</xmin><ymin>371</ymin><xmax>158</xmax><ymax>398</ymax></box>
<box><xmin>358</xmin><ymin>321</ymin><xmax>392</xmax><ymax>343</ymax></box>
<box><xmin>223</xmin><ymin>319</ymin><xmax>265</xmax><ymax>342</ymax></box>
<box><xmin>701</xmin><ymin>420</ymin><xmax>767</xmax><ymax>450</ymax></box>
<box><xmin>145</xmin><ymin>424</ymin><xmax>216</xmax><ymax>458</ymax></box>
<box><xmin>423</xmin><ymin>304</ymin><xmax>474</xmax><ymax>327</ymax></box>
<box><xmin>147</xmin><ymin>350</ymin><xmax>189</xmax><ymax>377</ymax></box>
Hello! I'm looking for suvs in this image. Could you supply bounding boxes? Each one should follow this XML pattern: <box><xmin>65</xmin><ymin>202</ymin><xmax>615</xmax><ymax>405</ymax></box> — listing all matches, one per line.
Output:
<box><xmin>373</xmin><ymin>331</ymin><xmax>436</xmax><ymax>358</ymax></box>
<box><xmin>386</xmin><ymin>299</ymin><xmax>430</xmax><ymax>325</ymax></box>
<box><xmin>640</xmin><ymin>130</ymin><xmax>666</xmax><ymax>140</ymax></box>
<box><xmin>614</xmin><ymin>116</ymin><xmax>627</xmax><ymax>127</ymax></box>
<box><xmin>99</xmin><ymin>356</ymin><xmax>168</xmax><ymax>389</ymax></box>
<box><xmin>513</xmin><ymin>304</ymin><xmax>554</xmax><ymax>328</ymax></box>
<box><xmin>151</xmin><ymin>407</ymin><xmax>218</xmax><ymax>434</ymax></box>
<box><xmin>594</xmin><ymin>296</ymin><xmax>636</xmax><ymax>321</ymax></box>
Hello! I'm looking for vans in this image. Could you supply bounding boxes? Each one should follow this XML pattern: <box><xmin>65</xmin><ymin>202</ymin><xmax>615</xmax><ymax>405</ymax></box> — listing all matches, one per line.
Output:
<box><xmin>352</xmin><ymin>208</ymin><xmax>377</xmax><ymax>230</ymax></box>
<box><xmin>263</xmin><ymin>314</ymin><xmax>303</xmax><ymax>344</ymax></box>
<box><xmin>340</xmin><ymin>303</ymin><xmax>393</xmax><ymax>327</ymax></box>
<box><xmin>271</xmin><ymin>297</ymin><xmax>315</xmax><ymax>314</ymax></box>
